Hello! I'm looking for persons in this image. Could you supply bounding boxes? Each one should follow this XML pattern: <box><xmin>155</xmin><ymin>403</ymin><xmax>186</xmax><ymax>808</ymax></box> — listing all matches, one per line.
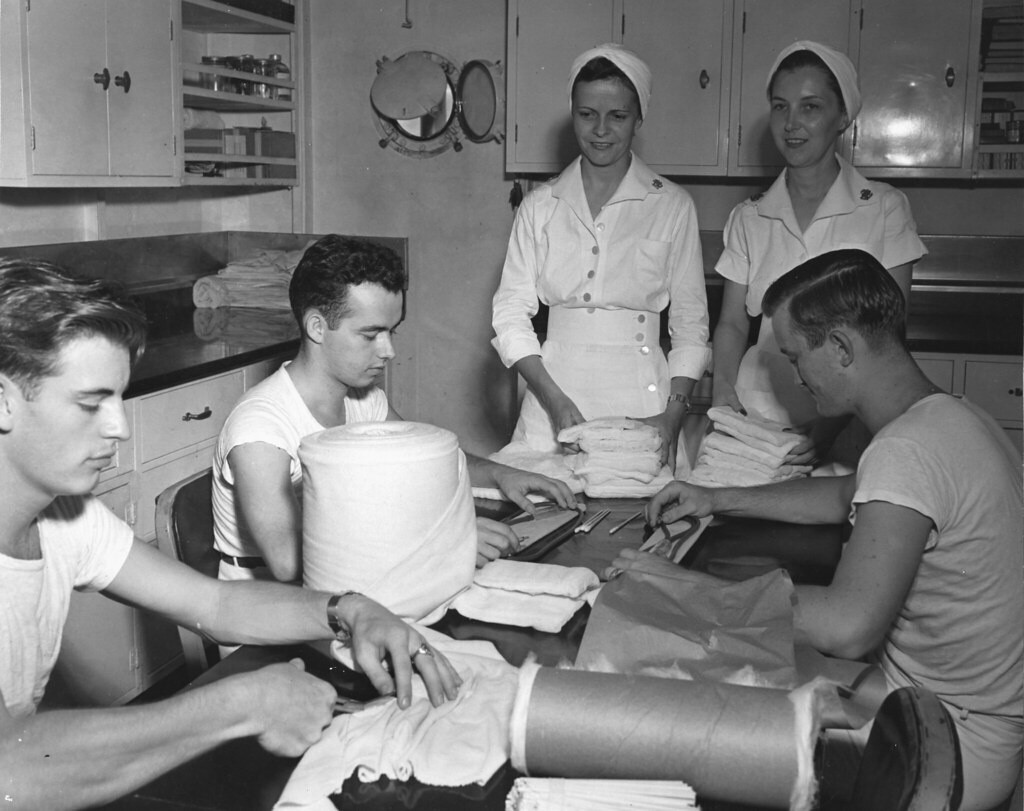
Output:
<box><xmin>717</xmin><ymin>40</ymin><xmax>928</xmax><ymax>467</ymax></box>
<box><xmin>0</xmin><ymin>254</ymin><xmax>461</xmax><ymax>811</ymax></box>
<box><xmin>211</xmin><ymin>235</ymin><xmax>578</xmax><ymax>663</ymax></box>
<box><xmin>488</xmin><ymin>44</ymin><xmax>710</xmax><ymax>479</ymax></box>
<box><xmin>594</xmin><ymin>248</ymin><xmax>1024</xmax><ymax>811</ymax></box>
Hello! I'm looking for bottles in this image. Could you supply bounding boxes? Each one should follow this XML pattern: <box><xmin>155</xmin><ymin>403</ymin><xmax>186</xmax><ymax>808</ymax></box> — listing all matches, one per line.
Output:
<box><xmin>202</xmin><ymin>54</ymin><xmax>292</xmax><ymax>100</ymax></box>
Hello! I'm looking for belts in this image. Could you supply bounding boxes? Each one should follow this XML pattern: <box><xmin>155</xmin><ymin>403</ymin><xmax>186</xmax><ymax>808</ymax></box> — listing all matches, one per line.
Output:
<box><xmin>218</xmin><ymin>550</ymin><xmax>265</xmax><ymax>569</ymax></box>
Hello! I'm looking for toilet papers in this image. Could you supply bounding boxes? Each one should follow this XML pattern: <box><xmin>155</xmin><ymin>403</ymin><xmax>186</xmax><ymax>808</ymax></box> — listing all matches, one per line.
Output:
<box><xmin>296</xmin><ymin>420</ymin><xmax>479</xmax><ymax>628</ymax></box>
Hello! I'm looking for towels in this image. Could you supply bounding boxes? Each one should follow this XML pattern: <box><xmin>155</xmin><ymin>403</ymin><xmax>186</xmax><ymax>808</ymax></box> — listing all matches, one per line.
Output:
<box><xmin>470</xmin><ymin>415</ymin><xmax>676</xmax><ymax>504</ymax></box>
<box><xmin>448</xmin><ymin>558</ymin><xmax>602</xmax><ymax>636</ymax></box>
<box><xmin>685</xmin><ymin>405</ymin><xmax>814</xmax><ymax>489</ymax></box>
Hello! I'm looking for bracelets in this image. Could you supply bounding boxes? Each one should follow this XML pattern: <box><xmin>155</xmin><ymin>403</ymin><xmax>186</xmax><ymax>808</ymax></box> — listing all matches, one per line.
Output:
<box><xmin>667</xmin><ymin>392</ymin><xmax>691</xmax><ymax>415</ymax></box>
<box><xmin>326</xmin><ymin>591</ymin><xmax>365</xmax><ymax>644</ymax></box>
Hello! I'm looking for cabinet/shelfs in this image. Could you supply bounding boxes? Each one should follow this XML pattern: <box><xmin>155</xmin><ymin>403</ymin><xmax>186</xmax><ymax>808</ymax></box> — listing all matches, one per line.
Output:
<box><xmin>503</xmin><ymin>0</ymin><xmax>1024</xmax><ymax>181</ymax></box>
<box><xmin>0</xmin><ymin>0</ymin><xmax>302</xmax><ymax>188</ymax></box>
<box><xmin>33</xmin><ymin>345</ymin><xmax>300</xmax><ymax>714</ymax></box>
<box><xmin>909</xmin><ymin>351</ymin><xmax>1023</xmax><ymax>458</ymax></box>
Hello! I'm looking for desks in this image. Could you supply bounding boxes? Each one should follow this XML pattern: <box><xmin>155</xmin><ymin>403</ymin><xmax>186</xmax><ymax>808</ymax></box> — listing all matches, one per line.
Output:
<box><xmin>86</xmin><ymin>488</ymin><xmax>856</xmax><ymax>810</ymax></box>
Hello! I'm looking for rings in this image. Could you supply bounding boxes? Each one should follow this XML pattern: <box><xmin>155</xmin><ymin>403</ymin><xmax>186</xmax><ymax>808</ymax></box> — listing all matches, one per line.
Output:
<box><xmin>412</xmin><ymin>643</ymin><xmax>435</xmax><ymax>663</ymax></box>
<box><xmin>609</xmin><ymin>567</ymin><xmax>622</xmax><ymax>581</ymax></box>
